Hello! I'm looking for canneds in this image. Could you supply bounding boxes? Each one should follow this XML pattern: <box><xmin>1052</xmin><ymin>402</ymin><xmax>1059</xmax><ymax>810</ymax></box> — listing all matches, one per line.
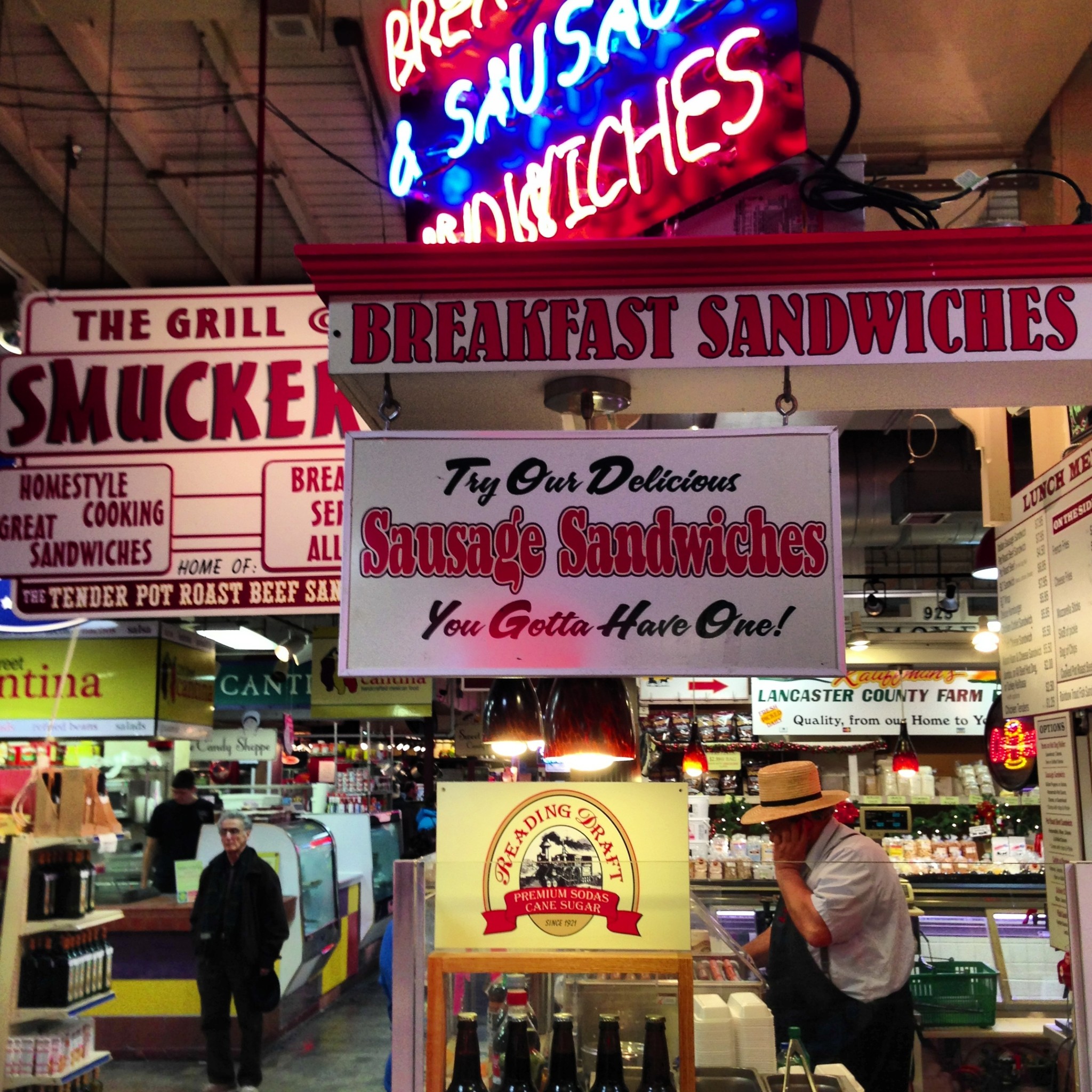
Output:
<box><xmin>337</xmin><ymin>769</ymin><xmax>369</xmax><ymax>792</ymax></box>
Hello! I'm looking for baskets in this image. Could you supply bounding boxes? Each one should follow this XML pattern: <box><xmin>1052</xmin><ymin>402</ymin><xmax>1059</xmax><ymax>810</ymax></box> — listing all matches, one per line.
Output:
<box><xmin>908</xmin><ymin>961</ymin><xmax>1000</xmax><ymax>1028</ymax></box>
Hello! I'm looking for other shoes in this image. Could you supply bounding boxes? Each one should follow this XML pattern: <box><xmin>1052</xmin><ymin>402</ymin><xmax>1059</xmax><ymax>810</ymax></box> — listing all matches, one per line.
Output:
<box><xmin>242</xmin><ymin>1084</ymin><xmax>258</xmax><ymax>1092</ymax></box>
<box><xmin>203</xmin><ymin>1080</ymin><xmax>237</xmax><ymax>1092</ymax></box>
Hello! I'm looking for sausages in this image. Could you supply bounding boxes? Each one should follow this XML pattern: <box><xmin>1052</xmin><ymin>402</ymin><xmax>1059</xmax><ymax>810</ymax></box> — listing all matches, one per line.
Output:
<box><xmin>596</xmin><ymin>958</ymin><xmax>740</xmax><ymax>981</ymax></box>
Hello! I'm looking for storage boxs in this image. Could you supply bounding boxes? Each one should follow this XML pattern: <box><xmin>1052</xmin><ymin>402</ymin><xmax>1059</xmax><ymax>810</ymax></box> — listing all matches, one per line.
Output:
<box><xmin>909</xmin><ymin>961</ymin><xmax>1001</xmax><ymax>1029</ymax></box>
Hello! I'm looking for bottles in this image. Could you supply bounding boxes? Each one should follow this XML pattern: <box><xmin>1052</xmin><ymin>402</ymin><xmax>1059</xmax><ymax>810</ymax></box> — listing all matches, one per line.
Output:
<box><xmin>4</xmin><ymin>1066</ymin><xmax>103</xmax><ymax>1092</ymax></box>
<box><xmin>541</xmin><ymin>1013</ymin><xmax>584</xmax><ymax>1092</ymax></box>
<box><xmin>487</xmin><ymin>970</ymin><xmax>543</xmax><ymax>1092</ymax></box>
<box><xmin>21</xmin><ymin>845</ymin><xmax>98</xmax><ymax>921</ymax></box>
<box><xmin>15</xmin><ymin>924</ymin><xmax>116</xmax><ymax>1009</ymax></box>
<box><xmin>634</xmin><ymin>1015</ymin><xmax>678</xmax><ymax>1092</ymax></box>
<box><xmin>587</xmin><ymin>1013</ymin><xmax>630</xmax><ymax>1092</ymax></box>
<box><xmin>448</xmin><ymin>1012</ymin><xmax>490</xmax><ymax>1092</ymax></box>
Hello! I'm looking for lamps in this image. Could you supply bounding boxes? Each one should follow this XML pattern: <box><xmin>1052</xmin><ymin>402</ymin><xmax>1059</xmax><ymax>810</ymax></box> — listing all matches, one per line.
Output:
<box><xmin>380</xmin><ymin>763</ymin><xmax>434</xmax><ymax>780</ymax></box>
<box><xmin>971</xmin><ymin>527</ymin><xmax>998</xmax><ymax>580</ymax></box>
<box><xmin>682</xmin><ymin>677</ymin><xmax>708</xmax><ymax>777</ymax></box>
<box><xmin>935</xmin><ymin>577</ymin><xmax>959</xmax><ymax>613</ymax></box>
<box><xmin>542</xmin><ymin>678</ymin><xmax>636</xmax><ymax>772</ymax></box>
<box><xmin>863</xmin><ymin>578</ymin><xmax>886</xmax><ymax>616</ymax></box>
<box><xmin>481</xmin><ymin>678</ymin><xmax>544</xmax><ymax>757</ymax></box>
<box><xmin>275</xmin><ymin>626</ymin><xmax>312</xmax><ymax>666</ymax></box>
<box><xmin>846</xmin><ymin>611</ymin><xmax>871</xmax><ymax>646</ymax></box>
<box><xmin>361</xmin><ymin>730</ymin><xmax>426</xmax><ymax>752</ymax></box>
<box><xmin>890</xmin><ymin>664</ymin><xmax>919</xmax><ymax>778</ymax></box>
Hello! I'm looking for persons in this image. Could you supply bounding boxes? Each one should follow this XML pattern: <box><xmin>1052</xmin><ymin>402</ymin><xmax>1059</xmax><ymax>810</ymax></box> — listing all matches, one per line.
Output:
<box><xmin>394</xmin><ymin>781</ymin><xmax>436</xmax><ymax>858</ymax></box>
<box><xmin>377</xmin><ymin>918</ymin><xmax>391</xmax><ymax>1092</ymax></box>
<box><xmin>190</xmin><ymin>812</ymin><xmax>290</xmax><ymax>1092</ymax></box>
<box><xmin>140</xmin><ymin>769</ymin><xmax>214</xmax><ymax>897</ymax></box>
<box><xmin>739</xmin><ymin>762</ymin><xmax>917</xmax><ymax>1092</ymax></box>
<box><xmin>209</xmin><ymin>761</ymin><xmax>240</xmax><ymax>795</ymax></box>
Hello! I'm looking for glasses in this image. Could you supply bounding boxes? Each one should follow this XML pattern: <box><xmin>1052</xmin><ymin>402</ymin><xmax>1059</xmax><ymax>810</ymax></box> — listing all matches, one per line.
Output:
<box><xmin>765</xmin><ymin>816</ymin><xmax>802</xmax><ymax>835</ymax></box>
<box><xmin>219</xmin><ymin>826</ymin><xmax>248</xmax><ymax>836</ymax></box>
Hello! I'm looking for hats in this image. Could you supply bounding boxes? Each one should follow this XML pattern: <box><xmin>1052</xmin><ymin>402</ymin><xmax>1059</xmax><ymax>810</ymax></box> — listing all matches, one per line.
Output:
<box><xmin>172</xmin><ymin>769</ymin><xmax>196</xmax><ymax>790</ymax></box>
<box><xmin>741</xmin><ymin>761</ymin><xmax>849</xmax><ymax>825</ymax></box>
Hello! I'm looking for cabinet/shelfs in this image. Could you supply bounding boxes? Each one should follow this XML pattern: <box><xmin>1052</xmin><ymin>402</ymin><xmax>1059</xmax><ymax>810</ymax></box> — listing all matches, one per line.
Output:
<box><xmin>0</xmin><ymin>837</ymin><xmax>125</xmax><ymax>1092</ymax></box>
<box><xmin>640</xmin><ymin>701</ymin><xmax>1040</xmax><ymax>831</ymax></box>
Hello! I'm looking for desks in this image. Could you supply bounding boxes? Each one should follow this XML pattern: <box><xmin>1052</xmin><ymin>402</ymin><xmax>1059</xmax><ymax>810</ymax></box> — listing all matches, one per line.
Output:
<box><xmin>913</xmin><ymin>1018</ymin><xmax>1073</xmax><ymax>1092</ymax></box>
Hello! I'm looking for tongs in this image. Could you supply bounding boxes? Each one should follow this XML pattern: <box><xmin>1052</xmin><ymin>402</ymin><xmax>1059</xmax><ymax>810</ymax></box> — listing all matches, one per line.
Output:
<box><xmin>779</xmin><ymin>1028</ymin><xmax>817</xmax><ymax>1092</ymax></box>
